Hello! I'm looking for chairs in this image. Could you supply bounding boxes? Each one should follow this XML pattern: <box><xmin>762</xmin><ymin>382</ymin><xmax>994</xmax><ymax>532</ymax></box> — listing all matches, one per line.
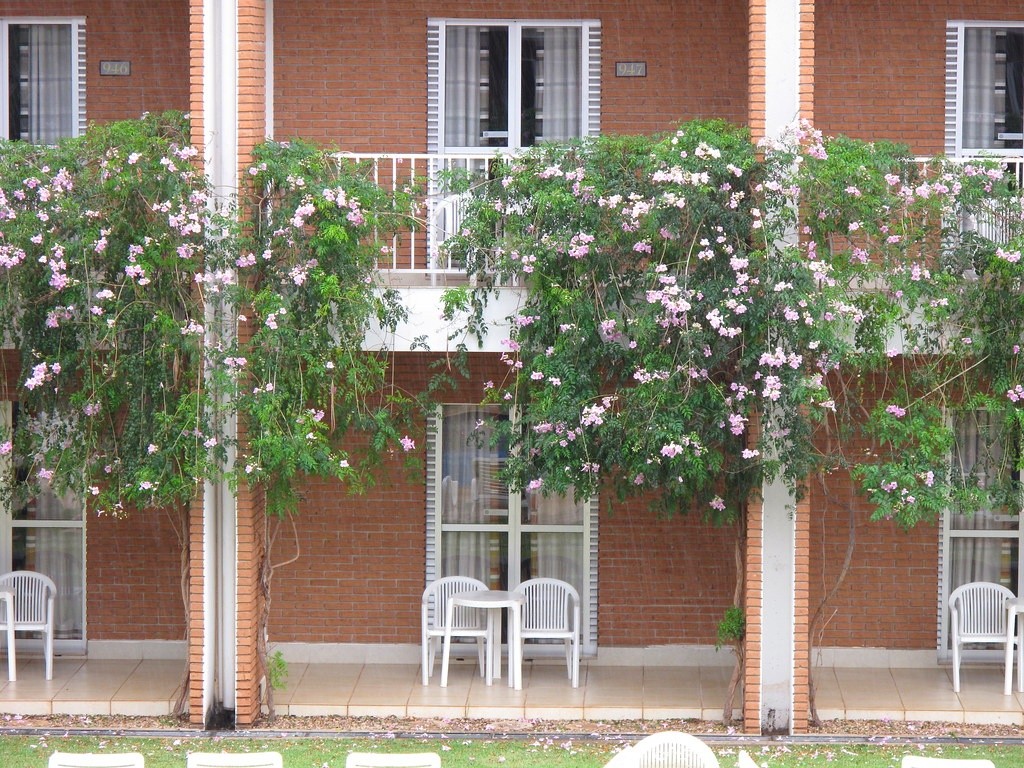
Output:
<box><xmin>430</xmin><ymin>189</ymin><xmax>504</xmax><ymax>284</ymax></box>
<box><xmin>507</xmin><ymin>577</ymin><xmax>580</xmax><ymax>689</ymax></box>
<box><xmin>421</xmin><ymin>576</ymin><xmax>494</xmax><ymax>686</ymax></box>
<box><xmin>48</xmin><ymin>750</ymin><xmax>443</xmax><ymax>767</ymax></box>
<box><xmin>0</xmin><ymin>570</ymin><xmax>57</xmax><ymax>680</ymax></box>
<box><xmin>949</xmin><ymin>582</ymin><xmax>1023</xmax><ymax>692</ymax></box>
<box><xmin>901</xmin><ymin>754</ymin><xmax>995</xmax><ymax>768</ymax></box>
<box><xmin>626</xmin><ymin>729</ymin><xmax>721</xmax><ymax>767</ymax></box>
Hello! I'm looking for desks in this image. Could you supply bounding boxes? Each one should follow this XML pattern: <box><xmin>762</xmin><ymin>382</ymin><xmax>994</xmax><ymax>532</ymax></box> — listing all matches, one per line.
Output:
<box><xmin>1003</xmin><ymin>597</ymin><xmax>1024</xmax><ymax>695</ymax></box>
<box><xmin>441</xmin><ymin>590</ymin><xmax>527</xmax><ymax>690</ymax></box>
